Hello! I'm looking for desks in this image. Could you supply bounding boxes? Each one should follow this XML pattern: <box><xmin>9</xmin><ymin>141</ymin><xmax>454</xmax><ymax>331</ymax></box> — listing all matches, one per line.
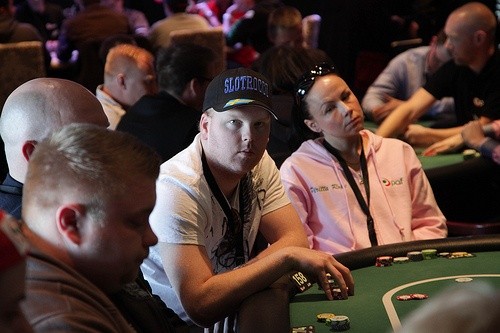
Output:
<box><xmin>288</xmin><ymin>231</ymin><xmax>500</xmax><ymax>332</ymax></box>
<box><xmin>364</xmin><ymin>118</ymin><xmax>482</xmax><ymax>178</ymax></box>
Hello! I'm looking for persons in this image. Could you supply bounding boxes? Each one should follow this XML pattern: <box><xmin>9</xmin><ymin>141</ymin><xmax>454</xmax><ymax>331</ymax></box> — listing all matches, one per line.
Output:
<box><xmin>288</xmin><ymin>71</ymin><xmax>447</xmax><ymax>241</ymax></box>
<box><xmin>254</xmin><ymin>45</ymin><xmax>312</xmax><ymax>100</ymax></box>
<box><xmin>249</xmin><ymin>7</ymin><xmax>400</xmax><ymax>144</ymax></box>
<box><xmin>88</xmin><ymin>42</ymin><xmax>166</xmax><ymax>114</ymax></box>
<box><xmin>0</xmin><ymin>127</ymin><xmax>182</xmax><ymax>333</ymax></box>
<box><xmin>142</xmin><ymin>67</ymin><xmax>357</xmax><ymax>329</ymax></box>
<box><xmin>0</xmin><ymin>75</ymin><xmax>110</xmax><ymax>217</ymax></box>
<box><xmin>361</xmin><ymin>3</ymin><xmax>500</xmax><ymax>164</ymax></box>
<box><xmin>385</xmin><ymin>0</ymin><xmax>448</xmax><ymax>57</ymax></box>
<box><xmin>0</xmin><ymin>0</ymin><xmax>273</xmax><ymax>66</ymax></box>
<box><xmin>123</xmin><ymin>45</ymin><xmax>217</xmax><ymax>164</ymax></box>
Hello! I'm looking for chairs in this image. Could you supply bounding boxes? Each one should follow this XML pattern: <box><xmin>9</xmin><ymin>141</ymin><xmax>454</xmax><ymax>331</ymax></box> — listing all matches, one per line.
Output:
<box><xmin>0</xmin><ymin>41</ymin><xmax>45</xmax><ymax>113</ymax></box>
<box><xmin>168</xmin><ymin>27</ymin><xmax>225</xmax><ymax>76</ymax></box>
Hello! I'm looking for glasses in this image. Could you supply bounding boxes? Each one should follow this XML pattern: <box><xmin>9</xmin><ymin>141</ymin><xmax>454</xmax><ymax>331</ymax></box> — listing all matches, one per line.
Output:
<box><xmin>296</xmin><ymin>61</ymin><xmax>336</xmax><ymax>106</ymax></box>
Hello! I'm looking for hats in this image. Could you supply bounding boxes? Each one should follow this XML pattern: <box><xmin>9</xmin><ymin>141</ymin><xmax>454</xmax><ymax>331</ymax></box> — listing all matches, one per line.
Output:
<box><xmin>202</xmin><ymin>67</ymin><xmax>279</xmax><ymax>120</ymax></box>
